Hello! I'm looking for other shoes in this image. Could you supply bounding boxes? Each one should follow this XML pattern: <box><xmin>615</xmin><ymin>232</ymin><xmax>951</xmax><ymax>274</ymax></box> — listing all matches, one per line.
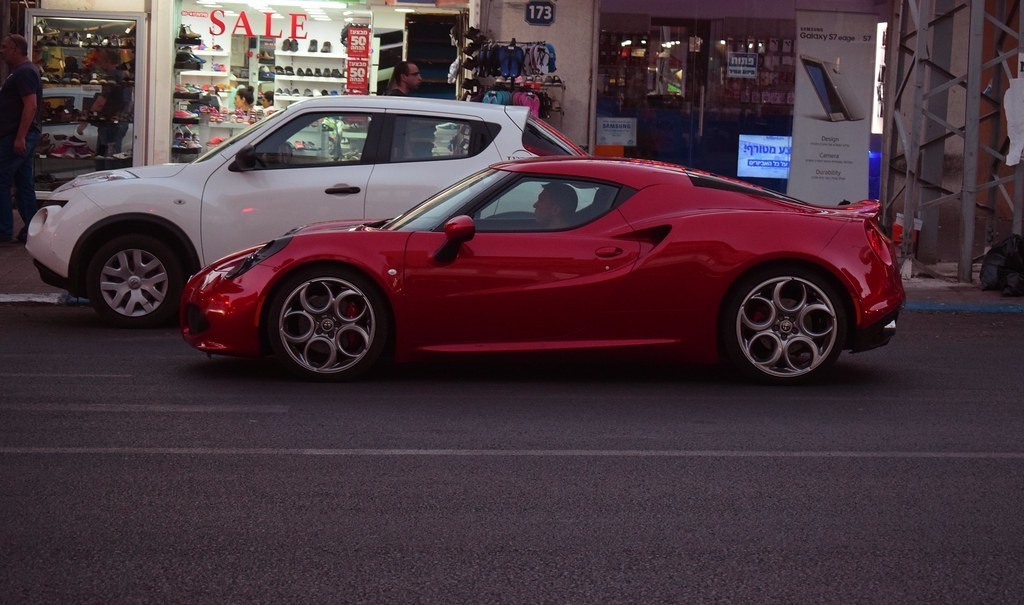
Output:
<box><xmin>171</xmin><ymin>23</ymin><xmax>345</xmax><ymax>155</ymax></box>
<box><xmin>16</xmin><ymin>232</ymin><xmax>28</xmax><ymax>241</ymax></box>
<box><xmin>31</xmin><ymin>23</ymin><xmax>135</xmax><ymax>189</ymax></box>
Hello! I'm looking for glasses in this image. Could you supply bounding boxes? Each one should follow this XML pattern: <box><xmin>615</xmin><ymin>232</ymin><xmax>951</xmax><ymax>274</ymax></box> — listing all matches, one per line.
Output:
<box><xmin>408</xmin><ymin>71</ymin><xmax>420</xmax><ymax>76</ymax></box>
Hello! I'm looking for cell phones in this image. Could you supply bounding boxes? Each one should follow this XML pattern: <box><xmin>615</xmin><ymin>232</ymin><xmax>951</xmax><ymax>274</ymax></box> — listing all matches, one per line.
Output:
<box><xmin>799</xmin><ymin>53</ymin><xmax>848</xmax><ymax>122</ymax></box>
<box><xmin>822</xmin><ymin>60</ymin><xmax>866</xmax><ymax>120</ymax></box>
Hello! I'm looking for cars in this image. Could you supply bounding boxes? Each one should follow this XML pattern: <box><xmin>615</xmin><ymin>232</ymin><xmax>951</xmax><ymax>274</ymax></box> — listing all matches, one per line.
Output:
<box><xmin>26</xmin><ymin>95</ymin><xmax>599</xmax><ymax>328</ymax></box>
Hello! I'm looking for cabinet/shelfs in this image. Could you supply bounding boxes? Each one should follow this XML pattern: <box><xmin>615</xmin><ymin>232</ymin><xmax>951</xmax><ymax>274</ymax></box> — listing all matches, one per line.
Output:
<box><xmin>32</xmin><ymin>42</ymin><xmax>134</xmax><ymax>162</ymax></box>
<box><xmin>171</xmin><ymin>36</ymin><xmax>349</xmax><ymax>161</ymax></box>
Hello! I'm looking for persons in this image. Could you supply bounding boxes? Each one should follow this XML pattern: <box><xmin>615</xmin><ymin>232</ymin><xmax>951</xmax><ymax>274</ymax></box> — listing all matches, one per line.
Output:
<box><xmin>33</xmin><ymin>44</ymin><xmax>45</xmax><ymax>70</ymax></box>
<box><xmin>77</xmin><ymin>51</ymin><xmax>133</xmax><ymax>170</ymax></box>
<box><xmin>261</xmin><ymin>91</ymin><xmax>273</xmax><ymax>110</ymax></box>
<box><xmin>0</xmin><ymin>35</ymin><xmax>42</xmax><ymax>241</ymax></box>
<box><xmin>532</xmin><ymin>181</ymin><xmax>579</xmax><ymax>229</ymax></box>
<box><xmin>385</xmin><ymin>61</ymin><xmax>423</xmax><ymax>161</ymax></box>
<box><xmin>234</xmin><ymin>86</ymin><xmax>255</xmax><ymax>109</ymax></box>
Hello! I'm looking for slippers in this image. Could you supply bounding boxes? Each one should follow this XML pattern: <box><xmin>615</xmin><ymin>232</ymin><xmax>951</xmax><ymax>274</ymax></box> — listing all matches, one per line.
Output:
<box><xmin>463</xmin><ymin>28</ymin><xmax>559</xmax><ymax>119</ymax></box>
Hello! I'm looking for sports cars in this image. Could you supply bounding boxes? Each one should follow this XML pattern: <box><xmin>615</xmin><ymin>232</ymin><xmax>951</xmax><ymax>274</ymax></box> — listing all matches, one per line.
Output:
<box><xmin>178</xmin><ymin>157</ymin><xmax>905</xmax><ymax>384</ymax></box>
